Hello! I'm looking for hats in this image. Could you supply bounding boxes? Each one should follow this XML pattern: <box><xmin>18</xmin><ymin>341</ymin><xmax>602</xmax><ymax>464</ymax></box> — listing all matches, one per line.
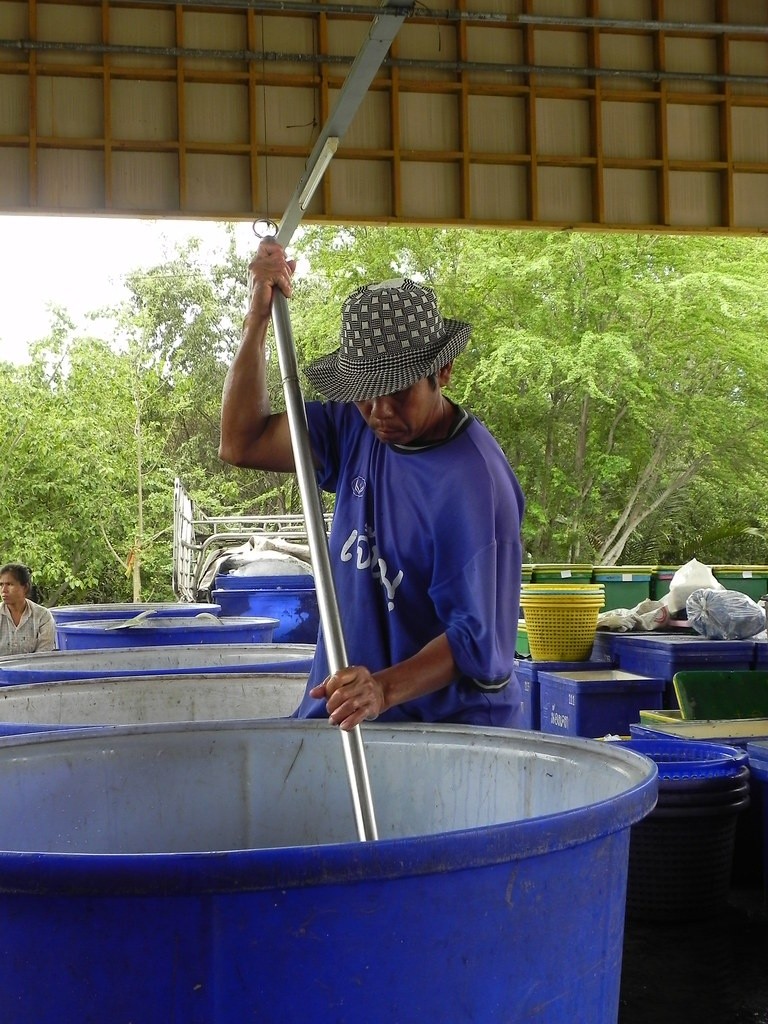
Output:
<box><xmin>301</xmin><ymin>276</ymin><xmax>473</xmax><ymax>403</ymax></box>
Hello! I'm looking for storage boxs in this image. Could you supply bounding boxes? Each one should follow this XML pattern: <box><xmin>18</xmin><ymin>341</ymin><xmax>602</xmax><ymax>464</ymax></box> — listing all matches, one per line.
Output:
<box><xmin>594</xmin><ymin>626</ymin><xmax>768</xmax><ymax>709</ymax></box>
<box><xmin>521</xmin><ymin>563</ymin><xmax>768</xmax><ymax>613</ymax></box>
<box><xmin>537</xmin><ymin>670</ymin><xmax>665</xmax><ymax>738</ymax></box>
<box><xmin>515</xmin><ymin>619</ymin><xmax>531</xmax><ymax>654</ymax></box>
<box><xmin>512</xmin><ymin>656</ymin><xmax>615</xmax><ymax>731</ymax></box>
<box><xmin>212</xmin><ymin>575</ymin><xmax>320</xmax><ymax>643</ymax></box>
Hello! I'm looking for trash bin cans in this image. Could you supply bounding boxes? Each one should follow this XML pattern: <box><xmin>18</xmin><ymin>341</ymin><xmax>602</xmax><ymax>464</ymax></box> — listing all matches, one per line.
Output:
<box><xmin>601</xmin><ymin>738</ymin><xmax>750</xmax><ymax>922</ymax></box>
<box><xmin>712</xmin><ymin>565</ymin><xmax>768</xmax><ymax>604</ymax></box>
<box><xmin>520</xmin><ymin>584</ymin><xmax>605</xmax><ymax>661</ymax></box>
<box><xmin>592</xmin><ymin>565</ymin><xmax>653</xmax><ymax>613</ymax></box>
<box><xmin>520</xmin><ymin>564</ymin><xmax>532</xmax><ymax>620</ymax></box>
<box><xmin>532</xmin><ymin>561</ymin><xmax>593</xmax><ymax>583</ymax></box>
<box><xmin>650</xmin><ymin>565</ymin><xmax>685</xmax><ymax>601</ymax></box>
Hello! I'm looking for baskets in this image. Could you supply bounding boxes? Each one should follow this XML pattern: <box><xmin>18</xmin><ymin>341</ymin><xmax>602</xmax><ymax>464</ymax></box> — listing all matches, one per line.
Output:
<box><xmin>520</xmin><ymin>583</ymin><xmax>605</xmax><ymax>661</ymax></box>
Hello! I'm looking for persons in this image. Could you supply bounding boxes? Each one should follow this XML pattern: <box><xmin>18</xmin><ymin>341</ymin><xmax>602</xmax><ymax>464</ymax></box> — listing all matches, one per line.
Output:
<box><xmin>0</xmin><ymin>564</ymin><xmax>56</xmax><ymax>656</ymax></box>
<box><xmin>217</xmin><ymin>243</ymin><xmax>526</xmax><ymax>731</ymax></box>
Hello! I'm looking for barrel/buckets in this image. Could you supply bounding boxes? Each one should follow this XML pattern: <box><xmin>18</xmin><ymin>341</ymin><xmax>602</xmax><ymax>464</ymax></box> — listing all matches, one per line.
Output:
<box><xmin>0</xmin><ymin>674</ymin><xmax>312</xmax><ymax>735</ymax></box>
<box><xmin>55</xmin><ymin>616</ymin><xmax>281</xmax><ymax>650</ymax></box>
<box><xmin>47</xmin><ymin>602</ymin><xmax>222</xmax><ymax>624</ymax></box>
<box><xmin>0</xmin><ymin>720</ymin><xmax>659</xmax><ymax>1023</ymax></box>
<box><xmin>0</xmin><ymin>643</ymin><xmax>317</xmax><ymax>688</ymax></box>
